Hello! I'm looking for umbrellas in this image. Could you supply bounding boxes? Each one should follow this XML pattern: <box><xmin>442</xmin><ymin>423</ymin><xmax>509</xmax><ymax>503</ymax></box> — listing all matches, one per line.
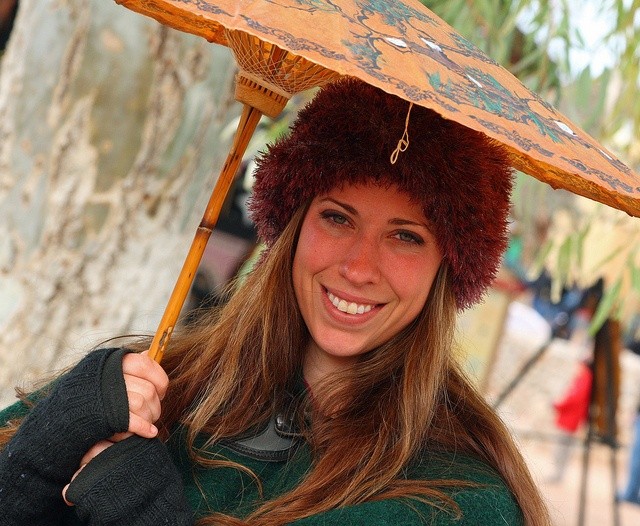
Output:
<box><xmin>115</xmin><ymin>0</ymin><xmax>640</xmax><ymax>364</ymax></box>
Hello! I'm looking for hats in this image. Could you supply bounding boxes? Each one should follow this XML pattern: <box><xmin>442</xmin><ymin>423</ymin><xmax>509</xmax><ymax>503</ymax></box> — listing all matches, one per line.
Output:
<box><xmin>247</xmin><ymin>75</ymin><xmax>516</xmax><ymax>310</ymax></box>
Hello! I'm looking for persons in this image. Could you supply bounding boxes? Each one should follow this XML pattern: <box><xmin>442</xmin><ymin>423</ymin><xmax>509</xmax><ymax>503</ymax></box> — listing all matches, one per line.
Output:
<box><xmin>614</xmin><ymin>397</ymin><xmax>640</xmax><ymax>526</ymax></box>
<box><xmin>1</xmin><ymin>79</ymin><xmax>553</xmax><ymax>524</ymax></box>
